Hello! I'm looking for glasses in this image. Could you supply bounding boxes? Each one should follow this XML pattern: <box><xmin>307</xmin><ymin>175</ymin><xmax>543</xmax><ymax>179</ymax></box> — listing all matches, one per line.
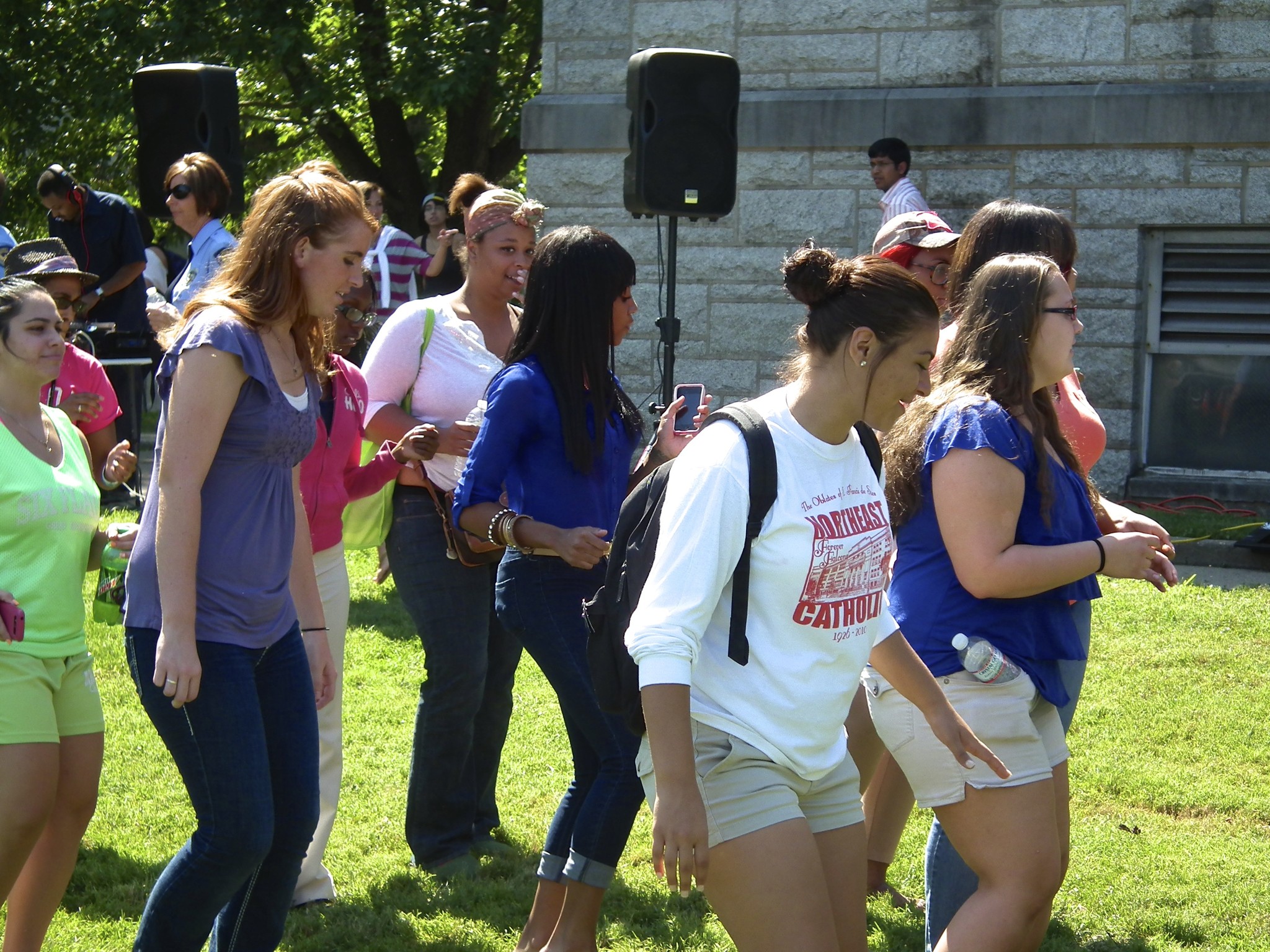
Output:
<box><xmin>910</xmin><ymin>262</ymin><xmax>951</xmax><ymax>285</ymax></box>
<box><xmin>51</xmin><ymin>297</ymin><xmax>84</xmax><ymax>314</ymax></box>
<box><xmin>159</xmin><ymin>183</ymin><xmax>193</xmax><ymax>202</ymax></box>
<box><xmin>338</xmin><ymin>305</ymin><xmax>378</xmax><ymax>327</ymax></box>
<box><xmin>1042</xmin><ymin>305</ymin><xmax>1078</xmax><ymax>320</ymax></box>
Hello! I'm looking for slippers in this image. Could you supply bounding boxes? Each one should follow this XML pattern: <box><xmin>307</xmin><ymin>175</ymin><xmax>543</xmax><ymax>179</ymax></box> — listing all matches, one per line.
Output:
<box><xmin>372</xmin><ymin>552</ymin><xmax>391</xmax><ymax>585</ymax></box>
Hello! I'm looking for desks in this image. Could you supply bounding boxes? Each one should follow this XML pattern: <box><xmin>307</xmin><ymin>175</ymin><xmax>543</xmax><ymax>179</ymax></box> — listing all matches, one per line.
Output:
<box><xmin>92</xmin><ymin>358</ymin><xmax>153</xmax><ymax>506</ymax></box>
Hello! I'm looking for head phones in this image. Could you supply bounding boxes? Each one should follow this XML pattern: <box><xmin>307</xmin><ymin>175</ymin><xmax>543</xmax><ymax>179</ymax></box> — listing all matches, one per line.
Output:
<box><xmin>48</xmin><ymin>164</ymin><xmax>86</xmax><ymax>205</ymax></box>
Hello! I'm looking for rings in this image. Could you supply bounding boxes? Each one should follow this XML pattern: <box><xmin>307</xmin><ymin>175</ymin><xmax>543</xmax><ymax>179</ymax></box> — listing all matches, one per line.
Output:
<box><xmin>77</xmin><ymin>403</ymin><xmax>82</xmax><ymax>412</ymax></box>
<box><xmin>165</xmin><ymin>679</ymin><xmax>177</xmax><ymax>684</ymax></box>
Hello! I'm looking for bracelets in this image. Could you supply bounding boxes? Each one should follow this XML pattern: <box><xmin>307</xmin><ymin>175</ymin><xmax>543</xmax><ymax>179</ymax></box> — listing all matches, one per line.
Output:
<box><xmin>1091</xmin><ymin>537</ymin><xmax>1106</xmax><ymax>575</ymax></box>
<box><xmin>101</xmin><ymin>465</ymin><xmax>120</xmax><ymax>486</ymax></box>
<box><xmin>488</xmin><ymin>509</ymin><xmax>536</xmax><ymax>554</ymax></box>
<box><xmin>301</xmin><ymin>627</ymin><xmax>330</xmax><ymax>632</ymax></box>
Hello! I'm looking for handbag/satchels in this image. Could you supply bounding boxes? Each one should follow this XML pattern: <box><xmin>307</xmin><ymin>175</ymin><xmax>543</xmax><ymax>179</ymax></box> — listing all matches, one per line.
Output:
<box><xmin>441</xmin><ymin>488</ymin><xmax>507</xmax><ymax>566</ymax></box>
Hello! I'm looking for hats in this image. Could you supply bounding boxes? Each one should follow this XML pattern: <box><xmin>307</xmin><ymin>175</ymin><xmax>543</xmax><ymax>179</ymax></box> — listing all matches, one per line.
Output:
<box><xmin>872</xmin><ymin>210</ymin><xmax>962</xmax><ymax>255</ymax></box>
<box><xmin>422</xmin><ymin>192</ymin><xmax>449</xmax><ymax>206</ymax></box>
<box><xmin>0</xmin><ymin>237</ymin><xmax>100</xmax><ymax>287</ymax></box>
<box><xmin>464</xmin><ymin>188</ymin><xmax>551</xmax><ymax>240</ymax></box>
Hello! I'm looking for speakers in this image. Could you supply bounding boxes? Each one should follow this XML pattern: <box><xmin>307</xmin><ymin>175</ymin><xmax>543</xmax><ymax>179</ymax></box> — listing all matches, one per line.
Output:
<box><xmin>622</xmin><ymin>47</ymin><xmax>740</xmax><ymax>221</ymax></box>
<box><xmin>134</xmin><ymin>62</ymin><xmax>246</xmax><ymax>219</ymax></box>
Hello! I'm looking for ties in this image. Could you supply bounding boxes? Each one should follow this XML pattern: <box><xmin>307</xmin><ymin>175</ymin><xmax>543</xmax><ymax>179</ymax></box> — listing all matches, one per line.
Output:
<box><xmin>164</xmin><ymin>244</ymin><xmax>194</xmax><ymax>303</ymax></box>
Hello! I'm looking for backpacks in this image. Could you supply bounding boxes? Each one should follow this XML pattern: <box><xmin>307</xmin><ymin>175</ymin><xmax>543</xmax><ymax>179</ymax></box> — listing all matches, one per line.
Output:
<box><xmin>581</xmin><ymin>400</ymin><xmax>779</xmax><ymax>739</ymax></box>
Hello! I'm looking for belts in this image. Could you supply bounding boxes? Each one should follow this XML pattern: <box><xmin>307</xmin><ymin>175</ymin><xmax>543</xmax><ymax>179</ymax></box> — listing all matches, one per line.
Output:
<box><xmin>524</xmin><ymin>541</ymin><xmax>613</xmax><ymax>557</ymax></box>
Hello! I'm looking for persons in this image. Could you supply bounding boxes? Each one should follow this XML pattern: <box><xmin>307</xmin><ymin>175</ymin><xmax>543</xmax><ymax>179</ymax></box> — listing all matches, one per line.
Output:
<box><xmin>623</xmin><ymin>239</ymin><xmax>1013</xmax><ymax>952</ymax></box>
<box><xmin>361</xmin><ymin>172</ymin><xmax>545</xmax><ymax>880</ymax></box>
<box><xmin>844</xmin><ymin>210</ymin><xmax>958</xmax><ymax>914</ymax></box>
<box><xmin>869</xmin><ymin>199</ymin><xmax>1108</xmax><ymax>952</ymax></box>
<box><xmin>0</xmin><ymin>152</ymin><xmax>469</xmax><ymax>952</ymax></box>
<box><xmin>860</xmin><ymin>255</ymin><xmax>1178</xmax><ymax>952</ymax></box>
<box><xmin>452</xmin><ymin>224</ymin><xmax>711</xmax><ymax>952</ymax></box>
<box><xmin>866</xmin><ymin>136</ymin><xmax>931</xmax><ymax>229</ymax></box>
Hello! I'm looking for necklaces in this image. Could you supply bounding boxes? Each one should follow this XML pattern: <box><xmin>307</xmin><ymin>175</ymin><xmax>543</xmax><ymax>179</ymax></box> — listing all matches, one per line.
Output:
<box><xmin>0</xmin><ymin>407</ymin><xmax>53</xmax><ymax>452</ymax></box>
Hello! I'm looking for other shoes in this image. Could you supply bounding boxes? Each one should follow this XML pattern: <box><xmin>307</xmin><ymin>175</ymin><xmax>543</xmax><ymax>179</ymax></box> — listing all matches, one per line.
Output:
<box><xmin>430</xmin><ymin>839</ymin><xmax>517</xmax><ymax>884</ymax></box>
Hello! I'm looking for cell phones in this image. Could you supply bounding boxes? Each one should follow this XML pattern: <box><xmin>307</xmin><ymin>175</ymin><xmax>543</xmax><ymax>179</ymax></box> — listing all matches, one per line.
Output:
<box><xmin>672</xmin><ymin>383</ymin><xmax>705</xmax><ymax>436</ymax></box>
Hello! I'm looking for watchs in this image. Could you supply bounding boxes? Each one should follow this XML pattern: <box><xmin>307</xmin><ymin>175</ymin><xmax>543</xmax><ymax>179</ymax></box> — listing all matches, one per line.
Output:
<box><xmin>94</xmin><ymin>285</ymin><xmax>106</xmax><ymax>299</ymax></box>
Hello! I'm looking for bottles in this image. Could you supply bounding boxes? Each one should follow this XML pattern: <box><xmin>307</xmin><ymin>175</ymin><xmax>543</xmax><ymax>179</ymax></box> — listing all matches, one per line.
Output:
<box><xmin>146</xmin><ymin>286</ymin><xmax>170</xmax><ymax>313</ymax></box>
<box><xmin>92</xmin><ymin>526</ymin><xmax>132</xmax><ymax>626</ymax></box>
<box><xmin>951</xmin><ymin>633</ymin><xmax>1020</xmax><ymax>684</ymax></box>
<box><xmin>454</xmin><ymin>399</ymin><xmax>487</xmax><ymax>478</ymax></box>
